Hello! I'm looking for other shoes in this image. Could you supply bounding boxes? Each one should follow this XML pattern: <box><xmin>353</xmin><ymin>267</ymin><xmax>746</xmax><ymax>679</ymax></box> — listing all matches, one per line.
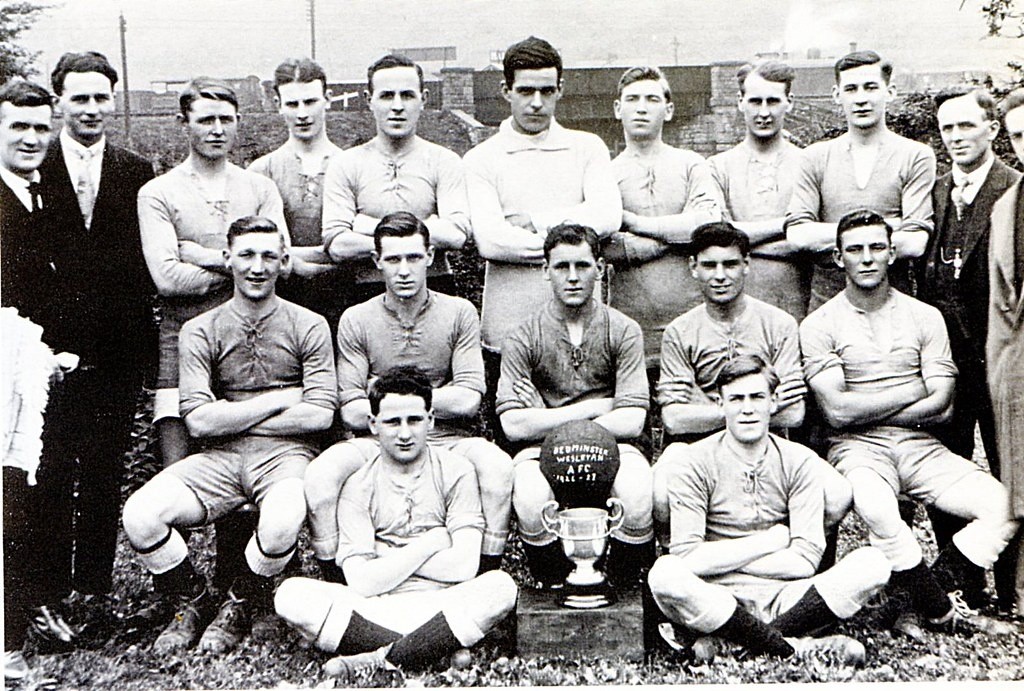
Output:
<box><xmin>26</xmin><ymin>595</ymin><xmax>99</xmax><ymax>650</ymax></box>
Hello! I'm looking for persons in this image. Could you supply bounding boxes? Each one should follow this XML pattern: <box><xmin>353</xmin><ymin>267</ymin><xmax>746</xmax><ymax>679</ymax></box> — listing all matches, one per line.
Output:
<box><xmin>2</xmin><ymin>38</ymin><xmax>1024</xmax><ymax>688</ymax></box>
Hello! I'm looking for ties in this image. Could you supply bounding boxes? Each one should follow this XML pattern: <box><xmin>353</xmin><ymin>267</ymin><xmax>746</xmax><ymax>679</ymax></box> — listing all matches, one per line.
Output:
<box><xmin>77</xmin><ymin>150</ymin><xmax>96</xmax><ymax>231</ymax></box>
<box><xmin>954</xmin><ymin>177</ymin><xmax>969</xmax><ymax>220</ymax></box>
<box><xmin>26</xmin><ymin>181</ymin><xmax>40</xmax><ymax>212</ymax></box>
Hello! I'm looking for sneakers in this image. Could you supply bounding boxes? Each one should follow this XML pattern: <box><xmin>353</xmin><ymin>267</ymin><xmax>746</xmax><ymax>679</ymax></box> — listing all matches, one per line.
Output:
<box><xmin>323</xmin><ymin>640</ymin><xmax>405</xmax><ymax>688</ymax></box>
<box><xmin>780</xmin><ymin>636</ymin><xmax>866</xmax><ymax>670</ymax></box>
<box><xmin>693</xmin><ymin>635</ymin><xmax>754</xmax><ymax>663</ymax></box>
<box><xmin>417</xmin><ymin>647</ymin><xmax>471</xmax><ymax>672</ymax></box>
<box><xmin>153</xmin><ymin>586</ymin><xmax>212</xmax><ymax>655</ymax></box>
<box><xmin>927</xmin><ymin>589</ymin><xmax>1015</xmax><ymax>638</ymax></box>
<box><xmin>198</xmin><ymin>579</ymin><xmax>250</xmax><ymax>656</ymax></box>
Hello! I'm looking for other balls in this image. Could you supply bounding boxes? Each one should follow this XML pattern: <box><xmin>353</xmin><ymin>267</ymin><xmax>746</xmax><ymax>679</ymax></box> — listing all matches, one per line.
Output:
<box><xmin>539</xmin><ymin>420</ymin><xmax>620</xmax><ymax>489</ymax></box>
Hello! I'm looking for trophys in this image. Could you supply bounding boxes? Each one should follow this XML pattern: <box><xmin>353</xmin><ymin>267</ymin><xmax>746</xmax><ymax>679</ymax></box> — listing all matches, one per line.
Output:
<box><xmin>540</xmin><ymin>497</ymin><xmax>625</xmax><ymax>611</ymax></box>
